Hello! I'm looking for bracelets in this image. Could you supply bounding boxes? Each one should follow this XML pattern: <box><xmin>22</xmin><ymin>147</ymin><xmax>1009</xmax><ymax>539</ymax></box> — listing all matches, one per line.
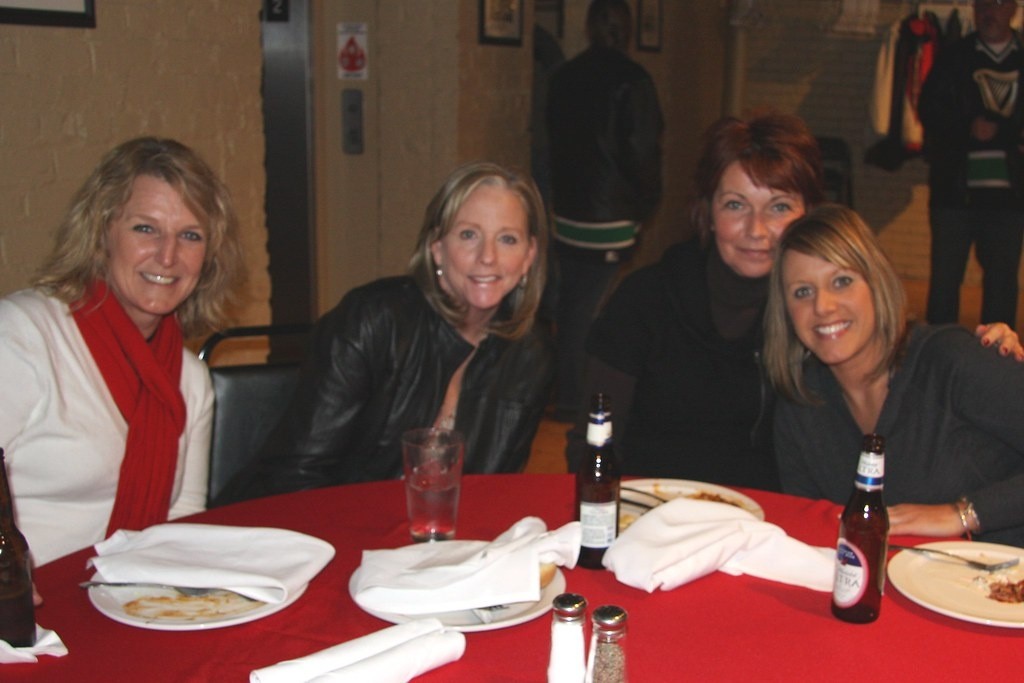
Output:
<box><xmin>953</xmin><ymin>497</ymin><xmax>980</xmax><ymax>534</ymax></box>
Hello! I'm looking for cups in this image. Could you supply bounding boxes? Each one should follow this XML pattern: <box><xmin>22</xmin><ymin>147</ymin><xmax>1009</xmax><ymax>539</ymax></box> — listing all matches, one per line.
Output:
<box><xmin>401</xmin><ymin>426</ymin><xmax>466</xmax><ymax>542</ymax></box>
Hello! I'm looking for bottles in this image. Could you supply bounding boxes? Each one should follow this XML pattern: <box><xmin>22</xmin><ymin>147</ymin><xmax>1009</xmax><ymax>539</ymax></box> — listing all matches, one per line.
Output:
<box><xmin>574</xmin><ymin>392</ymin><xmax>622</xmax><ymax>569</ymax></box>
<box><xmin>0</xmin><ymin>449</ymin><xmax>36</xmax><ymax>650</ymax></box>
<box><xmin>586</xmin><ymin>604</ymin><xmax>627</xmax><ymax>683</ymax></box>
<box><xmin>545</xmin><ymin>592</ymin><xmax>588</xmax><ymax>683</ymax></box>
<box><xmin>830</xmin><ymin>433</ymin><xmax>890</xmax><ymax>624</ymax></box>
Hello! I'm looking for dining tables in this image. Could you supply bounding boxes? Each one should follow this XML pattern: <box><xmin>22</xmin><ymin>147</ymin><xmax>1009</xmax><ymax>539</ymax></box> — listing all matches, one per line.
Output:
<box><xmin>0</xmin><ymin>475</ymin><xmax>1024</xmax><ymax>683</ymax></box>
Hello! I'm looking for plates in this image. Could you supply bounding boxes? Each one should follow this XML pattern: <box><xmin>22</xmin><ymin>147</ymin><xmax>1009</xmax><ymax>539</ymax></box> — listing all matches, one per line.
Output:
<box><xmin>349</xmin><ymin>540</ymin><xmax>567</xmax><ymax>632</ymax></box>
<box><xmin>616</xmin><ymin>477</ymin><xmax>764</xmax><ymax>546</ymax></box>
<box><xmin>87</xmin><ymin>571</ymin><xmax>307</xmax><ymax>631</ymax></box>
<box><xmin>888</xmin><ymin>541</ymin><xmax>1024</xmax><ymax>631</ymax></box>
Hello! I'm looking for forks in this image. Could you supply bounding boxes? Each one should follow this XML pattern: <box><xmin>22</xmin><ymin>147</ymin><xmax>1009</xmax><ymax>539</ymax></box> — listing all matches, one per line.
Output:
<box><xmin>886</xmin><ymin>542</ymin><xmax>1020</xmax><ymax>571</ymax></box>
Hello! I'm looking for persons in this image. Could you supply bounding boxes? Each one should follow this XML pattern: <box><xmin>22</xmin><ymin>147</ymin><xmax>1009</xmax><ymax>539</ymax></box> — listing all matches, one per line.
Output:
<box><xmin>565</xmin><ymin>108</ymin><xmax>1024</xmax><ymax>493</ymax></box>
<box><xmin>212</xmin><ymin>164</ymin><xmax>552</xmax><ymax>508</ymax></box>
<box><xmin>917</xmin><ymin>0</ymin><xmax>1024</xmax><ymax>336</ymax></box>
<box><xmin>546</xmin><ymin>0</ymin><xmax>666</xmax><ymax>423</ymax></box>
<box><xmin>758</xmin><ymin>205</ymin><xmax>1024</xmax><ymax>550</ymax></box>
<box><xmin>0</xmin><ymin>136</ymin><xmax>249</xmax><ymax>606</ymax></box>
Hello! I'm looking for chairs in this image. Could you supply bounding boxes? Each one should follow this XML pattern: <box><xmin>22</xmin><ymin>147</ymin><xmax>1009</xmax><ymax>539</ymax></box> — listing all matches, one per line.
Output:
<box><xmin>195</xmin><ymin>324</ymin><xmax>322</xmax><ymax>504</ymax></box>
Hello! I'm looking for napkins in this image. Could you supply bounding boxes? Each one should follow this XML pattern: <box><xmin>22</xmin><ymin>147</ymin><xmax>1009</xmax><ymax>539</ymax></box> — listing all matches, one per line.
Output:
<box><xmin>601</xmin><ymin>499</ymin><xmax>837</xmax><ymax>593</ymax></box>
<box><xmin>249</xmin><ymin>617</ymin><xmax>467</xmax><ymax>683</ymax></box>
<box><xmin>359</xmin><ymin>513</ymin><xmax>582</xmax><ymax>616</ymax></box>
<box><xmin>87</xmin><ymin>522</ymin><xmax>336</xmax><ymax>601</ymax></box>
<box><xmin>0</xmin><ymin>621</ymin><xmax>68</xmax><ymax>665</ymax></box>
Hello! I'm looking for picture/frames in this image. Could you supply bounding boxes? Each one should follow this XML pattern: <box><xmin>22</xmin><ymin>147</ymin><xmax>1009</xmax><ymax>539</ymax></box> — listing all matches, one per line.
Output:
<box><xmin>478</xmin><ymin>0</ymin><xmax>523</xmax><ymax>44</ymax></box>
<box><xmin>636</xmin><ymin>1</ymin><xmax>662</xmax><ymax>51</ymax></box>
<box><xmin>0</xmin><ymin>0</ymin><xmax>96</xmax><ymax>28</ymax></box>
